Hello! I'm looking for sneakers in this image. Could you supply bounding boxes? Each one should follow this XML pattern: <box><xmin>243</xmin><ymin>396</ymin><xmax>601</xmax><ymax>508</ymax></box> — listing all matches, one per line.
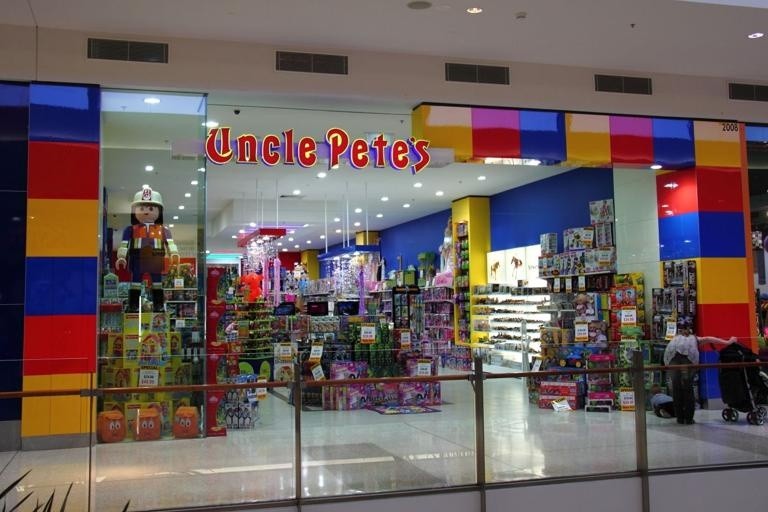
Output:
<box><xmin>677</xmin><ymin>418</ymin><xmax>695</xmax><ymax>424</ymax></box>
<box><xmin>660</xmin><ymin>409</ymin><xmax>671</xmax><ymax>418</ymax></box>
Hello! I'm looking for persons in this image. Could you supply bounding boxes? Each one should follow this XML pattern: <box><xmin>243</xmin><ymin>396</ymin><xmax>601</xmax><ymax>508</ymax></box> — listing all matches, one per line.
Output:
<box><xmin>647</xmin><ymin>384</ymin><xmax>674</xmax><ymax>419</ymax></box>
<box><xmin>659</xmin><ymin>329</ymin><xmax>738</xmax><ymax>424</ymax></box>
<box><xmin>115</xmin><ymin>184</ymin><xmax>181</xmax><ymax>312</ymax></box>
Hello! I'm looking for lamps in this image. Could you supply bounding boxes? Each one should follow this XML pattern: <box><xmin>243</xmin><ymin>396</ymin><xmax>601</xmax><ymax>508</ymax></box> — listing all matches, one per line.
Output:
<box><xmin>316</xmin><ymin>182</ymin><xmax>381</xmax><ymax>262</ymax></box>
<box><xmin>237</xmin><ymin>177</ymin><xmax>286</xmax><ymax>249</ymax></box>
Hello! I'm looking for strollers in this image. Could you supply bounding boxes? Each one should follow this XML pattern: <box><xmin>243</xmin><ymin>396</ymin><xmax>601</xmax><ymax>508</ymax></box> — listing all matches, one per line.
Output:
<box><xmin>710</xmin><ymin>342</ymin><xmax>768</xmax><ymax>426</ymax></box>
